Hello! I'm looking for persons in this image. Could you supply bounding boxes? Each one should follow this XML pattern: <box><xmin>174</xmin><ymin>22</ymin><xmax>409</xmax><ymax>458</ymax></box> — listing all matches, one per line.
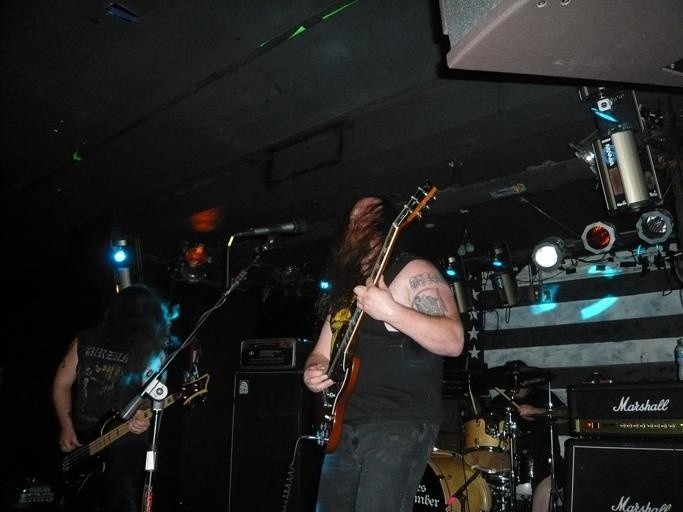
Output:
<box><xmin>49</xmin><ymin>285</ymin><xmax>171</xmax><ymax>511</ymax></box>
<box><xmin>488</xmin><ymin>361</ymin><xmax>569</xmax><ymax>511</ymax></box>
<box><xmin>302</xmin><ymin>198</ymin><xmax>465</xmax><ymax>512</ymax></box>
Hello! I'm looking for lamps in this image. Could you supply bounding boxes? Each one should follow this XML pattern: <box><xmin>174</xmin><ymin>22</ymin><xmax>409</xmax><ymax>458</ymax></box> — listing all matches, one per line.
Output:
<box><xmin>180</xmin><ymin>243</ymin><xmax>210</xmax><ymax>285</ymax></box>
<box><xmin>637</xmin><ymin>208</ymin><xmax>674</xmax><ymax>248</ymax></box>
<box><xmin>580</xmin><ymin>216</ymin><xmax>617</xmax><ymax>252</ymax></box>
<box><xmin>532</xmin><ymin>234</ymin><xmax>566</xmax><ymax>277</ymax></box>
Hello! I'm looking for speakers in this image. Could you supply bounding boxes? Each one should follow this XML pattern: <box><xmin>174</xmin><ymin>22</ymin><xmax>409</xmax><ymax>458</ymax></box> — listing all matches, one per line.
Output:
<box><xmin>228</xmin><ymin>370</ymin><xmax>325</xmax><ymax>512</ymax></box>
<box><xmin>564</xmin><ymin>438</ymin><xmax>683</xmax><ymax>512</ymax></box>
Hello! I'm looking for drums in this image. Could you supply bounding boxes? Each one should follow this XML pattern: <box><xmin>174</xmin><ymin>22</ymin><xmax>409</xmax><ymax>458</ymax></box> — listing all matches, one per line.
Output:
<box><xmin>463</xmin><ymin>415</ymin><xmax>522</xmax><ymax>474</ymax></box>
<box><xmin>415</xmin><ymin>445</ymin><xmax>493</xmax><ymax>512</ymax></box>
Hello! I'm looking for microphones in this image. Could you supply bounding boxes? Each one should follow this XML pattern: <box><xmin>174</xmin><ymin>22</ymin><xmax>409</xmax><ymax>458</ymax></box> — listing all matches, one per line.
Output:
<box><xmin>510</xmin><ymin>362</ymin><xmax>521</xmax><ymax>393</ymax></box>
<box><xmin>228</xmin><ymin>216</ymin><xmax>309</xmax><ymax>239</ymax></box>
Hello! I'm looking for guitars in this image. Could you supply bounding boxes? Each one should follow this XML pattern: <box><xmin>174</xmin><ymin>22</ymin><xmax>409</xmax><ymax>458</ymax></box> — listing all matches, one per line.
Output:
<box><xmin>314</xmin><ymin>180</ymin><xmax>443</xmax><ymax>453</ymax></box>
<box><xmin>51</xmin><ymin>372</ymin><xmax>212</xmax><ymax>498</ymax></box>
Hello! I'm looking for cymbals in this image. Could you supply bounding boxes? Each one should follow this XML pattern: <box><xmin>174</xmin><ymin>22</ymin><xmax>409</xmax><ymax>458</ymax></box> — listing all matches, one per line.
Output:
<box><xmin>478</xmin><ymin>361</ymin><xmax>554</xmax><ymax>389</ymax></box>
